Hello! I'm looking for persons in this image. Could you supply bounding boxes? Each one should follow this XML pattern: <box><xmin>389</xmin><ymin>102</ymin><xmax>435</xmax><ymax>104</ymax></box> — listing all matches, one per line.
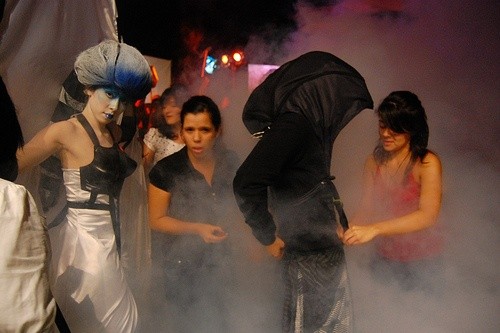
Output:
<box><xmin>14</xmin><ymin>38</ymin><xmax>148</xmax><ymax>333</ymax></box>
<box><xmin>148</xmin><ymin>95</ymin><xmax>240</xmax><ymax>305</ymax></box>
<box><xmin>0</xmin><ymin>178</ymin><xmax>58</xmax><ymax>333</ymax></box>
<box><xmin>142</xmin><ymin>84</ymin><xmax>189</xmax><ymax>187</ymax></box>
<box><xmin>233</xmin><ymin>51</ymin><xmax>375</xmax><ymax>333</ymax></box>
<box><xmin>339</xmin><ymin>90</ymin><xmax>442</xmax><ymax>262</ymax></box>
